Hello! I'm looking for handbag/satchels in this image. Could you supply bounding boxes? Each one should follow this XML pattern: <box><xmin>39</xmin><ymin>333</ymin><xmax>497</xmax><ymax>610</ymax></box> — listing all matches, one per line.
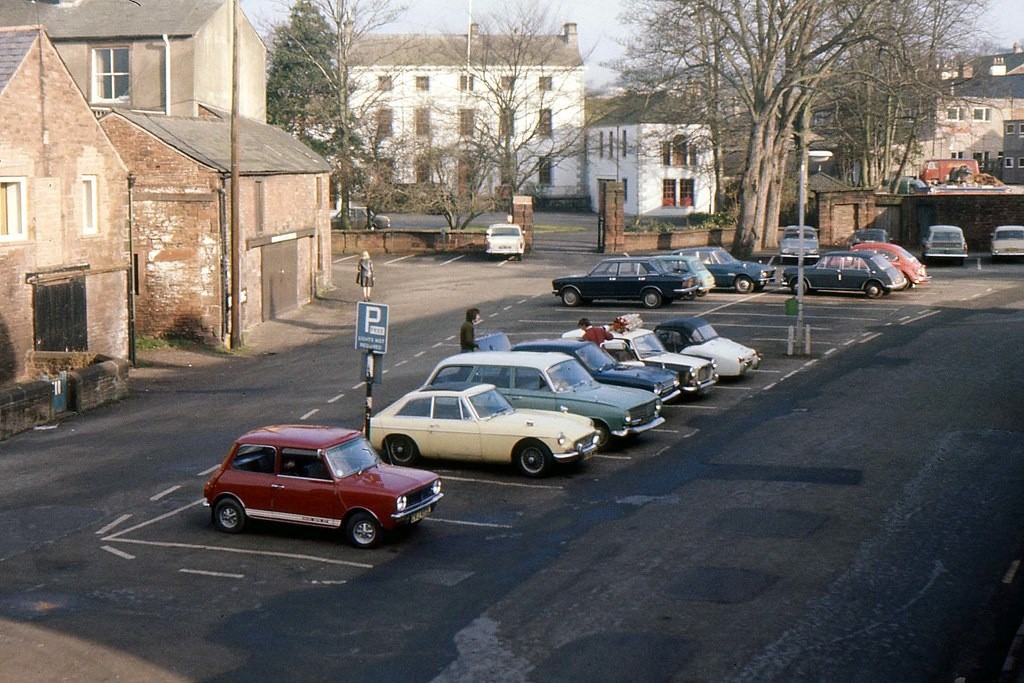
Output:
<box><xmin>356</xmin><ymin>272</ymin><xmax>361</xmax><ymax>283</ymax></box>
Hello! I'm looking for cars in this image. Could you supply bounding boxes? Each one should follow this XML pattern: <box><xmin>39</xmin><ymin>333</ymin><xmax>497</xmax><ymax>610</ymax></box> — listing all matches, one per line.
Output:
<box><xmin>989</xmin><ymin>224</ymin><xmax>1024</xmax><ymax>260</ymax></box>
<box><xmin>848</xmin><ymin>228</ymin><xmax>891</xmax><ymax>255</ymax></box>
<box><xmin>473</xmin><ymin>331</ymin><xmax>682</xmax><ymax>407</ymax></box>
<box><xmin>635</xmin><ymin>253</ymin><xmax>717</xmax><ymax>300</ymax></box>
<box><xmin>550</xmin><ymin>257</ymin><xmax>702</xmax><ymax>310</ymax></box>
<box><xmin>358</xmin><ymin>381</ymin><xmax>604</xmax><ymax>479</ymax></box>
<box><xmin>668</xmin><ymin>245</ymin><xmax>777</xmax><ymax>294</ymax></box>
<box><xmin>560</xmin><ymin>311</ymin><xmax>719</xmax><ymax>399</ymax></box>
<box><xmin>417</xmin><ymin>349</ymin><xmax>667</xmax><ymax>454</ymax></box>
<box><xmin>651</xmin><ymin>316</ymin><xmax>763</xmax><ymax>379</ymax></box>
<box><xmin>823</xmin><ymin>240</ymin><xmax>932</xmax><ymax>291</ymax></box>
<box><xmin>331</xmin><ymin>206</ymin><xmax>391</xmax><ymax>230</ymax></box>
<box><xmin>484</xmin><ymin>223</ymin><xmax>527</xmax><ymax>261</ymax></box>
<box><xmin>777</xmin><ymin>224</ymin><xmax>820</xmax><ymax>265</ymax></box>
<box><xmin>780</xmin><ymin>249</ymin><xmax>908</xmax><ymax>299</ymax></box>
<box><xmin>202</xmin><ymin>424</ymin><xmax>445</xmax><ymax>550</ymax></box>
<box><xmin>920</xmin><ymin>224</ymin><xmax>969</xmax><ymax>266</ymax></box>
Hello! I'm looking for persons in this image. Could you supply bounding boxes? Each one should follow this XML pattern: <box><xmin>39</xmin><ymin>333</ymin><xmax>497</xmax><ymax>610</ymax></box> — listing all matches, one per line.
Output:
<box><xmin>358</xmin><ymin>251</ymin><xmax>373</xmax><ymax>301</ymax></box>
<box><xmin>578</xmin><ymin>318</ymin><xmax>613</xmax><ymax>349</ymax></box>
<box><xmin>460</xmin><ymin>309</ymin><xmax>481</xmax><ymax>352</ymax></box>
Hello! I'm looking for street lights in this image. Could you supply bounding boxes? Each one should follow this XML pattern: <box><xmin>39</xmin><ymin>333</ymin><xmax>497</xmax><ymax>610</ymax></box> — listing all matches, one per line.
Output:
<box><xmin>794</xmin><ymin>149</ymin><xmax>832</xmax><ymax>357</ymax></box>
